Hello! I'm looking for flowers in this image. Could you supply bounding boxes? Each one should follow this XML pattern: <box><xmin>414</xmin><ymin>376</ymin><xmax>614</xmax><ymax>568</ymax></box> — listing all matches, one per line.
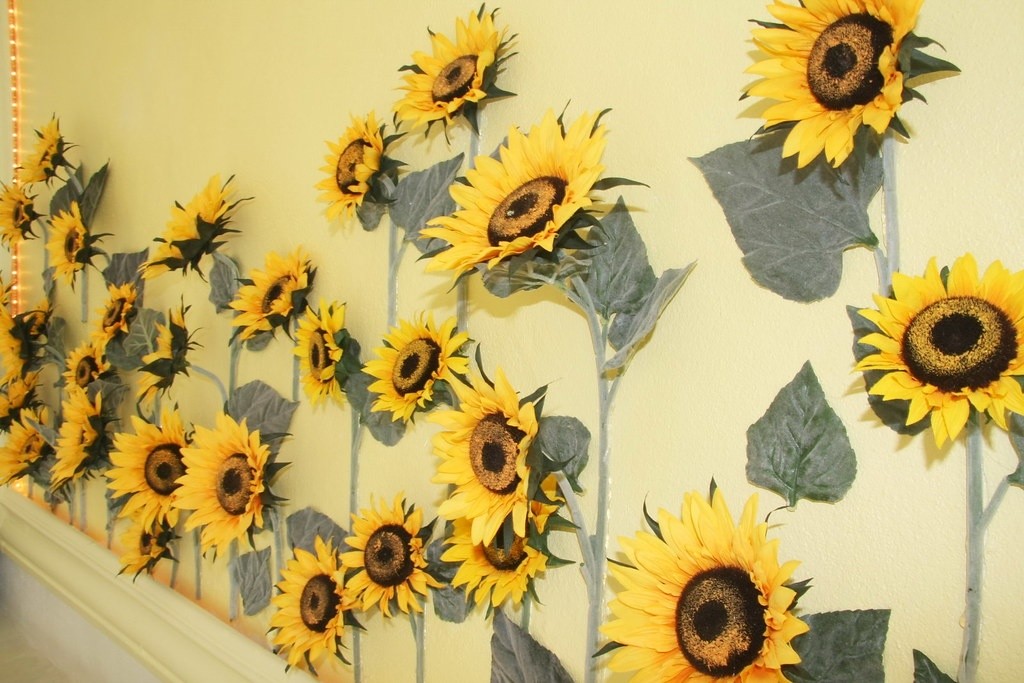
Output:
<box><xmin>0</xmin><ymin>0</ymin><xmax>1024</xmax><ymax>683</ymax></box>
